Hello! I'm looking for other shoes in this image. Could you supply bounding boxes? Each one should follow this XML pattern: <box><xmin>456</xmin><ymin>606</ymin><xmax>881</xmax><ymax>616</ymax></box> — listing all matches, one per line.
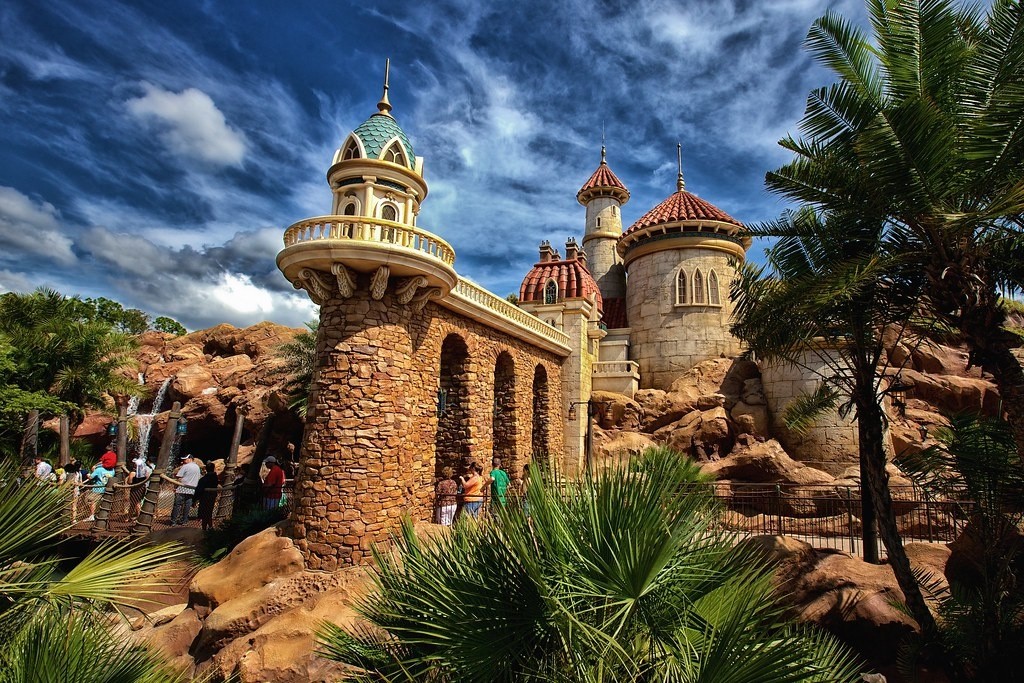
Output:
<box><xmin>83</xmin><ymin>515</ymin><xmax>95</xmax><ymax>522</ymax></box>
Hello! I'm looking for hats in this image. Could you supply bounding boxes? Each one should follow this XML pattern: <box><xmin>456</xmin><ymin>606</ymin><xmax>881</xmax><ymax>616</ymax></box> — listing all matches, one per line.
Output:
<box><xmin>181</xmin><ymin>454</ymin><xmax>193</xmax><ymax>459</ymax></box>
<box><xmin>263</xmin><ymin>456</ymin><xmax>276</xmax><ymax>462</ymax></box>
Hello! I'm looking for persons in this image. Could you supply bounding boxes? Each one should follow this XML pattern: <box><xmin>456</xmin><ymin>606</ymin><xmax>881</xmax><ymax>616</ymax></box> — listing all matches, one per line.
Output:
<box><xmin>8</xmin><ymin>455</ymin><xmax>92</xmax><ymax>523</ymax></box>
<box><xmin>435</xmin><ymin>466</ymin><xmax>458</xmax><ymax>526</ymax></box>
<box><xmin>262</xmin><ymin>456</ymin><xmax>283</xmax><ymax>510</ymax></box>
<box><xmin>191</xmin><ymin>463</ymin><xmax>218</xmax><ymax>532</ymax></box>
<box><xmin>123</xmin><ymin>458</ymin><xmax>149</xmax><ymax>523</ymax></box>
<box><xmin>459</xmin><ymin>461</ymin><xmax>484</xmax><ymax>523</ymax></box>
<box><xmin>480</xmin><ymin>457</ymin><xmax>512</xmax><ymax>511</ymax></box>
<box><xmin>277</xmin><ymin>457</ymin><xmax>298</xmax><ymax>478</ymax></box>
<box><xmin>231</xmin><ymin>462</ymin><xmax>250</xmax><ymax>518</ymax></box>
<box><xmin>234</xmin><ymin>466</ymin><xmax>244</xmax><ymax>484</ymax></box>
<box><xmin>91</xmin><ymin>445</ymin><xmax>117</xmax><ymax>471</ymax></box>
<box><xmin>520</xmin><ymin>463</ymin><xmax>534</xmax><ymax>518</ymax></box>
<box><xmin>145</xmin><ymin>458</ymin><xmax>157</xmax><ymax>487</ymax></box>
<box><xmin>83</xmin><ymin>465</ymin><xmax>116</xmax><ymax>522</ymax></box>
<box><xmin>169</xmin><ymin>453</ymin><xmax>201</xmax><ymax>527</ymax></box>
